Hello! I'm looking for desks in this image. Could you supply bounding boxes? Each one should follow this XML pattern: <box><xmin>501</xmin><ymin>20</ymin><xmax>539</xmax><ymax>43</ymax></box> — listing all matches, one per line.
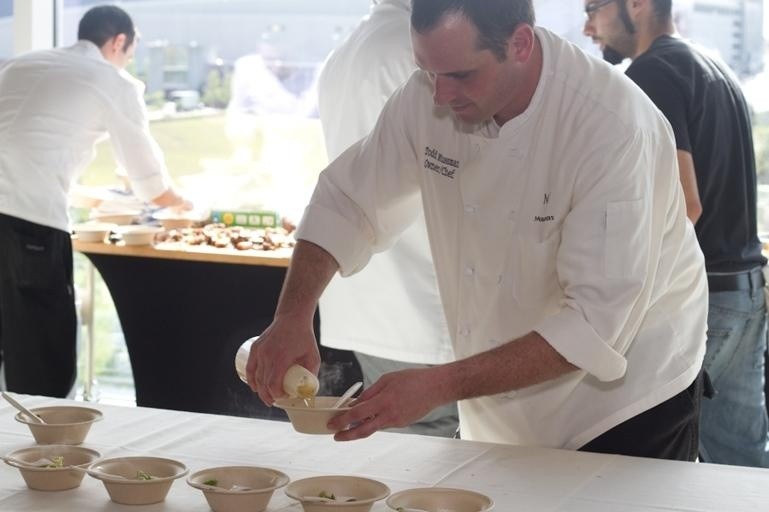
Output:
<box><xmin>64</xmin><ymin>209</ymin><xmax>342</xmax><ymax>424</ymax></box>
<box><xmin>0</xmin><ymin>391</ymin><xmax>769</xmax><ymax>512</ymax></box>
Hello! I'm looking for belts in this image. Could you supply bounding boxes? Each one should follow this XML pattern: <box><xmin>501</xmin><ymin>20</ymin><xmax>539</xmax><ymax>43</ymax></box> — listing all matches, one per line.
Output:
<box><xmin>709</xmin><ymin>270</ymin><xmax>765</xmax><ymax>291</ymax></box>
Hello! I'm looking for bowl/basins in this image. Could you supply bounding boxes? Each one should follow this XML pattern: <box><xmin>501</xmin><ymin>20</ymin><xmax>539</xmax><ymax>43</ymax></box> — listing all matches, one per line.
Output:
<box><xmin>384</xmin><ymin>487</ymin><xmax>498</xmax><ymax>512</ymax></box>
<box><xmin>71</xmin><ymin>209</ymin><xmax>196</xmax><ymax>248</ymax></box>
<box><xmin>282</xmin><ymin>475</ymin><xmax>391</xmax><ymax>511</ymax></box>
<box><xmin>86</xmin><ymin>456</ymin><xmax>189</xmax><ymax>504</ymax></box>
<box><xmin>186</xmin><ymin>462</ymin><xmax>291</xmax><ymax>511</ymax></box>
<box><xmin>1</xmin><ymin>444</ymin><xmax>101</xmax><ymax>492</ymax></box>
<box><xmin>266</xmin><ymin>395</ymin><xmax>366</xmax><ymax>434</ymax></box>
<box><xmin>15</xmin><ymin>408</ymin><xmax>104</xmax><ymax>445</ymax></box>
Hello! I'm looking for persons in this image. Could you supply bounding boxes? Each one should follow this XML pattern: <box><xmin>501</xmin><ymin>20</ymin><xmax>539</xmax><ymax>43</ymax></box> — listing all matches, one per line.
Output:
<box><xmin>313</xmin><ymin>0</ymin><xmax>461</xmax><ymax>439</ymax></box>
<box><xmin>244</xmin><ymin>0</ymin><xmax>709</xmax><ymax>462</ymax></box>
<box><xmin>582</xmin><ymin>0</ymin><xmax>769</xmax><ymax>468</ymax></box>
<box><xmin>1</xmin><ymin>5</ymin><xmax>197</xmax><ymax>398</ymax></box>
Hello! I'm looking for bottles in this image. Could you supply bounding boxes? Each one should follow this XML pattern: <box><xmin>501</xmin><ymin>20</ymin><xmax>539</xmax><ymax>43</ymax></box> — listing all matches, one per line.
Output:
<box><xmin>236</xmin><ymin>331</ymin><xmax>320</xmax><ymax>398</ymax></box>
